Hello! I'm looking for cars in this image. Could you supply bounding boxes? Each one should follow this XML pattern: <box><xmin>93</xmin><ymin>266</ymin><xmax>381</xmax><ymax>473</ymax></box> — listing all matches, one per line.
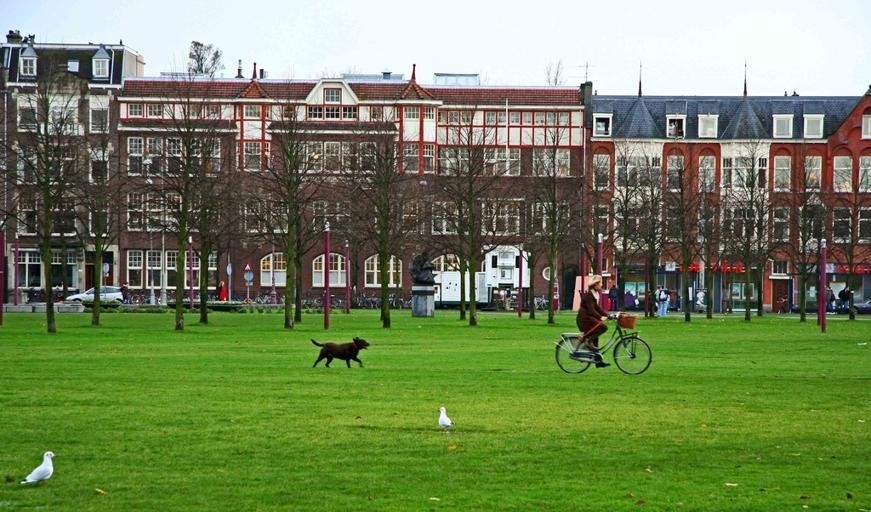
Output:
<box><xmin>65</xmin><ymin>285</ymin><xmax>125</xmax><ymax>308</ymax></box>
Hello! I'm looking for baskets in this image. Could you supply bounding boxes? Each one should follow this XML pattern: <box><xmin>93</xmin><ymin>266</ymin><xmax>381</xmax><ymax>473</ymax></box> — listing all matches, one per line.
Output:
<box><xmin>620</xmin><ymin>312</ymin><xmax>635</xmax><ymax>328</ymax></box>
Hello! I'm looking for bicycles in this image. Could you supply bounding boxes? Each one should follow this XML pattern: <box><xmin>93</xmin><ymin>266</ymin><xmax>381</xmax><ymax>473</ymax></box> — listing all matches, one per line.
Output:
<box><xmin>554</xmin><ymin>311</ymin><xmax>653</xmax><ymax>375</ymax></box>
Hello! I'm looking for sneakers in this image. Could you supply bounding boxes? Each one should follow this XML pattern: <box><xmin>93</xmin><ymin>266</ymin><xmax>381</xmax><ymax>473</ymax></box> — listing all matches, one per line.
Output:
<box><xmin>587</xmin><ymin>344</ymin><xmax>597</xmax><ymax>350</ymax></box>
<box><xmin>596</xmin><ymin>362</ymin><xmax>610</xmax><ymax>368</ymax></box>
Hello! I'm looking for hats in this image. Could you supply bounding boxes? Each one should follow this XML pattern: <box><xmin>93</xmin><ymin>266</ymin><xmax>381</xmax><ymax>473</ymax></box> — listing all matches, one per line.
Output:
<box><xmin>588</xmin><ymin>275</ymin><xmax>602</xmax><ymax>286</ymax></box>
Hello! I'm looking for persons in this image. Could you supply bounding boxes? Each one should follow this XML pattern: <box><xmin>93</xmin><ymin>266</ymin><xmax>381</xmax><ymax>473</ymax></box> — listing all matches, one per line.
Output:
<box><xmin>575</xmin><ymin>274</ymin><xmax>622</xmax><ymax>369</ymax></box>
<box><xmin>119</xmin><ymin>282</ymin><xmax>130</xmax><ymax>304</ymax></box>
<box><xmin>775</xmin><ymin>283</ymin><xmax>850</xmax><ymax>316</ymax></box>
<box><xmin>213</xmin><ymin>281</ymin><xmax>226</xmax><ymax>302</ymax></box>
<box><xmin>607</xmin><ymin>282</ymin><xmax>680</xmax><ymax>318</ymax></box>
<box><xmin>696</xmin><ymin>291</ymin><xmax>707</xmax><ymax>306</ymax></box>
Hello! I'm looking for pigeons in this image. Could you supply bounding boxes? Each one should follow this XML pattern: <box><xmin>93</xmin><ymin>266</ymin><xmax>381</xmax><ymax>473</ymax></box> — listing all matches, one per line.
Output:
<box><xmin>16</xmin><ymin>450</ymin><xmax>55</xmax><ymax>490</ymax></box>
<box><xmin>436</xmin><ymin>405</ymin><xmax>454</xmax><ymax>434</ymax></box>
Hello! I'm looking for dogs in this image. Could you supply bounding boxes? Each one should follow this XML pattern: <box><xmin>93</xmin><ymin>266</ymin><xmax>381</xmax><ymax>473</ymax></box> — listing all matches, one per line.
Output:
<box><xmin>308</xmin><ymin>335</ymin><xmax>370</xmax><ymax>369</ymax></box>
